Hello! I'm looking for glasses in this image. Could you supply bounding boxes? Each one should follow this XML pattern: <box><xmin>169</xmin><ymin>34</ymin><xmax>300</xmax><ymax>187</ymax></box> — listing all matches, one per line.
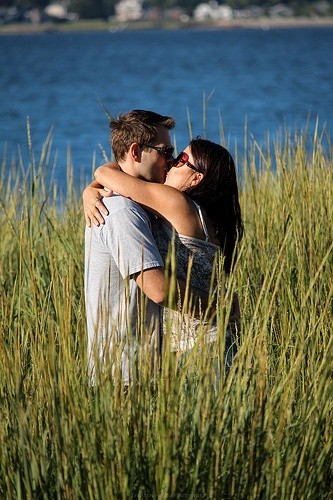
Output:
<box><xmin>143</xmin><ymin>144</ymin><xmax>174</xmax><ymax>160</ymax></box>
<box><xmin>173</xmin><ymin>152</ymin><xmax>200</xmax><ymax>172</ymax></box>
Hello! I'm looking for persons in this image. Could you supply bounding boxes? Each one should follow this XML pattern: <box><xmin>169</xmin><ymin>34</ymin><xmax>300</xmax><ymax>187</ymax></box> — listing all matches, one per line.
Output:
<box><xmin>82</xmin><ymin>110</ymin><xmax>177</xmax><ymax>396</ymax></box>
<box><xmin>82</xmin><ymin>138</ymin><xmax>247</xmax><ymax>369</ymax></box>
